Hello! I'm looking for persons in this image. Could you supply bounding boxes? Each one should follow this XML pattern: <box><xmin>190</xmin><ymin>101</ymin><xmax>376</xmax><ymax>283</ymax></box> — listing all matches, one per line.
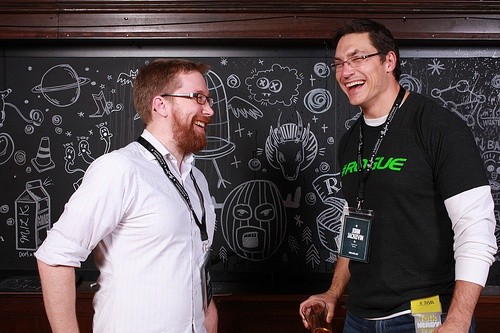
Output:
<box><xmin>300</xmin><ymin>19</ymin><xmax>498</xmax><ymax>333</ymax></box>
<box><xmin>33</xmin><ymin>58</ymin><xmax>220</xmax><ymax>333</ymax></box>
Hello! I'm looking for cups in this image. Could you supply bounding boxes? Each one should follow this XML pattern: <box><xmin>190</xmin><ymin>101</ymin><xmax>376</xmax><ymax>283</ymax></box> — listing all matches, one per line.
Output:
<box><xmin>301</xmin><ymin>299</ymin><xmax>333</xmax><ymax>333</ymax></box>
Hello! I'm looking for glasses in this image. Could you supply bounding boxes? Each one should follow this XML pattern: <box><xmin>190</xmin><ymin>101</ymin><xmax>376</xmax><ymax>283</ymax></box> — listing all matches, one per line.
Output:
<box><xmin>153</xmin><ymin>92</ymin><xmax>213</xmax><ymax>112</ymax></box>
<box><xmin>330</xmin><ymin>51</ymin><xmax>388</xmax><ymax>73</ymax></box>
